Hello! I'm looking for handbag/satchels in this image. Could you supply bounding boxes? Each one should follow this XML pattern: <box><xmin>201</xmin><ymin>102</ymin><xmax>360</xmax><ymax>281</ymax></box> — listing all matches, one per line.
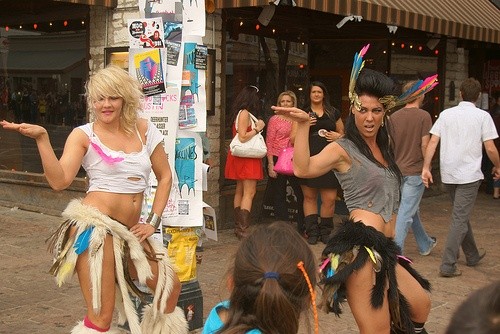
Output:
<box><xmin>230</xmin><ymin>109</ymin><xmax>267</xmax><ymax>158</ymax></box>
<box><xmin>273</xmin><ymin>146</ymin><xmax>295</xmax><ymax>175</ymax></box>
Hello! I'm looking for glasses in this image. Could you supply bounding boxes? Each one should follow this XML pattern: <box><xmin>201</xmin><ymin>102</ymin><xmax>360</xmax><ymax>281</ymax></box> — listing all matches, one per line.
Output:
<box><xmin>250</xmin><ymin>85</ymin><xmax>259</xmax><ymax>93</ymax></box>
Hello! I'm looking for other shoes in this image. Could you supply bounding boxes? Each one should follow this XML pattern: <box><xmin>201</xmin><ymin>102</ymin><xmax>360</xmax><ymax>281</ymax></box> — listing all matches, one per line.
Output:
<box><xmin>419</xmin><ymin>236</ymin><xmax>438</xmax><ymax>255</ymax></box>
<box><xmin>467</xmin><ymin>247</ymin><xmax>486</xmax><ymax>266</ymax></box>
<box><xmin>441</xmin><ymin>269</ymin><xmax>463</xmax><ymax>276</ymax></box>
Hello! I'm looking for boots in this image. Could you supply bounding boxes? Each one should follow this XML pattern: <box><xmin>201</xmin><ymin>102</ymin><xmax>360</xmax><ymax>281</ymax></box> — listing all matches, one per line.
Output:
<box><xmin>319</xmin><ymin>217</ymin><xmax>334</xmax><ymax>243</ymax></box>
<box><xmin>295</xmin><ymin>212</ymin><xmax>305</xmax><ymax>234</ymax></box>
<box><xmin>237</xmin><ymin>209</ymin><xmax>252</xmax><ymax>240</ymax></box>
<box><xmin>234</xmin><ymin>206</ymin><xmax>240</xmax><ymax>236</ymax></box>
<box><xmin>303</xmin><ymin>214</ymin><xmax>320</xmax><ymax>244</ymax></box>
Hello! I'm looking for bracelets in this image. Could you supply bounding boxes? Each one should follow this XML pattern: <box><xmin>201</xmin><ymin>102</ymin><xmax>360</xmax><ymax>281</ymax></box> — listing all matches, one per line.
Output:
<box><xmin>145</xmin><ymin>212</ymin><xmax>162</xmax><ymax>229</ymax></box>
<box><xmin>252</xmin><ymin>126</ymin><xmax>259</xmax><ymax>135</ymax></box>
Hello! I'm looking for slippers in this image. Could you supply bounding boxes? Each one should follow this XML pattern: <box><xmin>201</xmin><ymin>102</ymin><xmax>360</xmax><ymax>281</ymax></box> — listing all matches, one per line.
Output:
<box><xmin>494</xmin><ymin>195</ymin><xmax>500</xmax><ymax>200</ymax></box>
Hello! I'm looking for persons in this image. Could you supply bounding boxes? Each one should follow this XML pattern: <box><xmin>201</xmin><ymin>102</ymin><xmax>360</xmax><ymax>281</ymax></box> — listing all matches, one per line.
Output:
<box><xmin>482</xmin><ymin>91</ymin><xmax>500</xmax><ymax>200</ymax></box>
<box><xmin>384</xmin><ymin>78</ymin><xmax>437</xmax><ymax>257</ymax></box>
<box><xmin>202</xmin><ymin>220</ymin><xmax>317</xmax><ymax>334</ymax></box>
<box><xmin>0</xmin><ymin>66</ymin><xmax>183</xmax><ymax>334</ymax></box>
<box><xmin>443</xmin><ymin>281</ymin><xmax>500</xmax><ymax>334</ymax></box>
<box><xmin>269</xmin><ymin>66</ymin><xmax>432</xmax><ymax>334</ymax></box>
<box><xmin>227</xmin><ymin>84</ymin><xmax>267</xmax><ymax>239</ymax></box>
<box><xmin>420</xmin><ymin>78</ymin><xmax>500</xmax><ymax>277</ymax></box>
<box><xmin>290</xmin><ymin>81</ymin><xmax>346</xmax><ymax>244</ymax></box>
<box><xmin>8</xmin><ymin>87</ymin><xmax>87</xmax><ymax>124</ymax></box>
<box><xmin>264</xmin><ymin>90</ymin><xmax>310</xmax><ymax>241</ymax></box>
